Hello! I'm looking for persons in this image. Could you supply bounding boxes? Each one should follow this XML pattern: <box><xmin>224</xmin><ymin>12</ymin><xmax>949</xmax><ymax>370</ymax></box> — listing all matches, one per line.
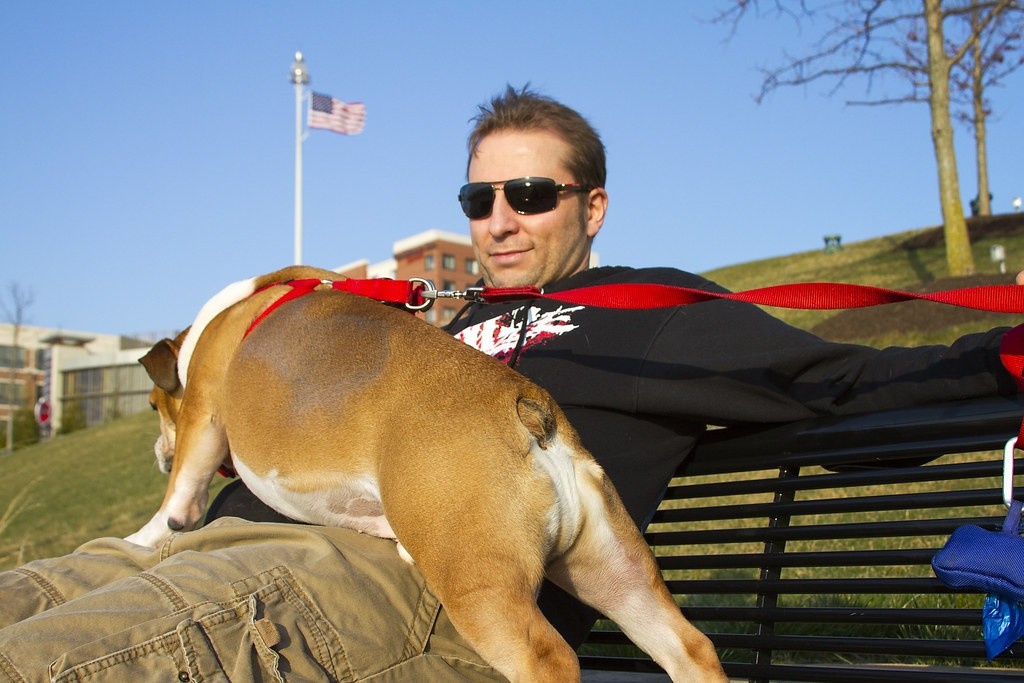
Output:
<box><xmin>0</xmin><ymin>91</ymin><xmax>1024</xmax><ymax>683</ymax></box>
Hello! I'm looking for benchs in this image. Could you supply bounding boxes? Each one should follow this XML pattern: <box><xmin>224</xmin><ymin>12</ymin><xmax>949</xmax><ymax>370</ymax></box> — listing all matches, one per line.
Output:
<box><xmin>575</xmin><ymin>391</ymin><xmax>1024</xmax><ymax>683</ymax></box>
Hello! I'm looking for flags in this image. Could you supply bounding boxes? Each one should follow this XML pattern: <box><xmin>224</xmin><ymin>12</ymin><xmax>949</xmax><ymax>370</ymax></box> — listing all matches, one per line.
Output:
<box><xmin>308</xmin><ymin>90</ymin><xmax>364</xmax><ymax>136</ymax></box>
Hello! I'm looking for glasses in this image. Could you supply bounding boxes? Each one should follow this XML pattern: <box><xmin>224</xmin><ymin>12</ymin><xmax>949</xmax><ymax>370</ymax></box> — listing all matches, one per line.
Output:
<box><xmin>457</xmin><ymin>177</ymin><xmax>594</xmax><ymax>220</ymax></box>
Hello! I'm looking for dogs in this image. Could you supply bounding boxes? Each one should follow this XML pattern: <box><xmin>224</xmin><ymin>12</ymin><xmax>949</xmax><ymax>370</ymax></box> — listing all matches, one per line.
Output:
<box><xmin>135</xmin><ymin>284</ymin><xmax>734</xmax><ymax>683</ymax></box>
